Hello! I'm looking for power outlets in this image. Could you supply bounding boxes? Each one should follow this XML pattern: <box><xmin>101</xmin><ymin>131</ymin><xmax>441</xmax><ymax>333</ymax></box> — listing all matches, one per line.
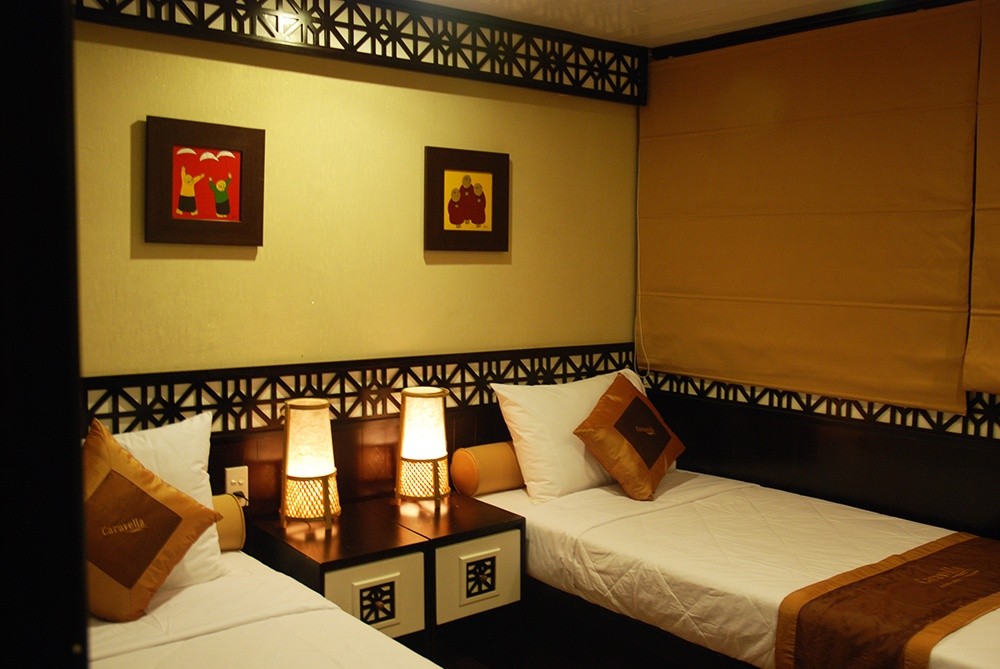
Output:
<box><xmin>224</xmin><ymin>465</ymin><xmax>249</xmax><ymax>507</ymax></box>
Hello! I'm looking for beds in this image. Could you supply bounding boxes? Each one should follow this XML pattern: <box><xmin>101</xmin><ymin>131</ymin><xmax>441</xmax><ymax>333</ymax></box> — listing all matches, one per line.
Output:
<box><xmin>86</xmin><ymin>550</ymin><xmax>444</xmax><ymax>669</ymax></box>
<box><xmin>473</xmin><ymin>467</ymin><xmax>1000</xmax><ymax>669</ymax></box>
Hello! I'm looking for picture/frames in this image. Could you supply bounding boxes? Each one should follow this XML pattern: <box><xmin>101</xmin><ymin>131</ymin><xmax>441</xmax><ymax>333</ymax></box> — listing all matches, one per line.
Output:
<box><xmin>424</xmin><ymin>146</ymin><xmax>510</xmax><ymax>249</ymax></box>
<box><xmin>144</xmin><ymin>114</ymin><xmax>266</xmax><ymax>245</ymax></box>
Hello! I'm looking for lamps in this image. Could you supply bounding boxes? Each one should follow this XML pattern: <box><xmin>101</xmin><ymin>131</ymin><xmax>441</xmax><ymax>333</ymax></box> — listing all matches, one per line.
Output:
<box><xmin>276</xmin><ymin>398</ymin><xmax>343</xmax><ymax>531</ymax></box>
<box><xmin>390</xmin><ymin>385</ymin><xmax>454</xmax><ymax>517</ymax></box>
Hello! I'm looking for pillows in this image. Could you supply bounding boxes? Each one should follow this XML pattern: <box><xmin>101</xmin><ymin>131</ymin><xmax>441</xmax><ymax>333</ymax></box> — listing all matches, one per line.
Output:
<box><xmin>450</xmin><ymin>441</ymin><xmax>525</xmax><ymax>498</ymax></box>
<box><xmin>213</xmin><ymin>493</ymin><xmax>246</xmax><ymax>551</ymax></box>
<box><xmin>81</xmin><ymin>409</ymin><xmax>227</xmax><ymax>590</ymax></box>
<box><xmin>81</xmin><ymin>416</ymin><xmax>225</xmax><ymax>624</ymax></box>
<box><xmin>489</xmin><ymin>367</ymin><xmax>676</xmax><ymax>504</ymax></box>
<box><xmin>573</xmin><ymin>372</ymin><xmax>685</xmax><ymax>501</ymax></box>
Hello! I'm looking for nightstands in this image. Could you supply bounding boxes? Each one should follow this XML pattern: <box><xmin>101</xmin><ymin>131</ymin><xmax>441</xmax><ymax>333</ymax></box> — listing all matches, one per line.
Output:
<box><xmin>248</xmin><ymin>489</ymin><xmax>528</xmax><ymax>640</ymax></box>
